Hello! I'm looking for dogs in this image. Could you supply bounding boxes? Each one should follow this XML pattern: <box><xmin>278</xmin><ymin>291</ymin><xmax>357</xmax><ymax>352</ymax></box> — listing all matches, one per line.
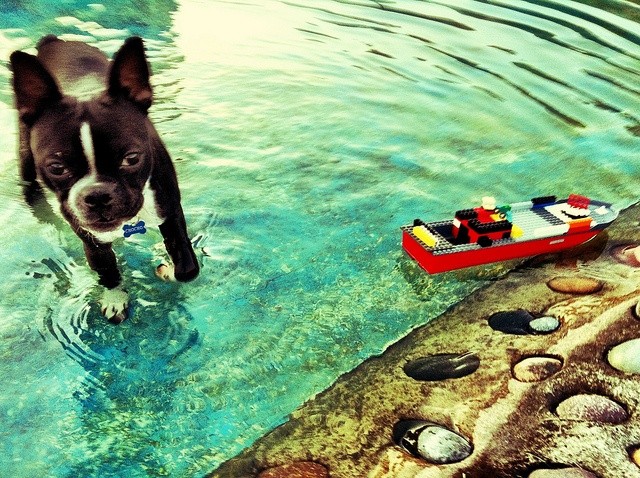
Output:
<box><xmin>0</xmin><ymin>34</ymin><xmax>200</xmax><ymax>326</ymax></box>
